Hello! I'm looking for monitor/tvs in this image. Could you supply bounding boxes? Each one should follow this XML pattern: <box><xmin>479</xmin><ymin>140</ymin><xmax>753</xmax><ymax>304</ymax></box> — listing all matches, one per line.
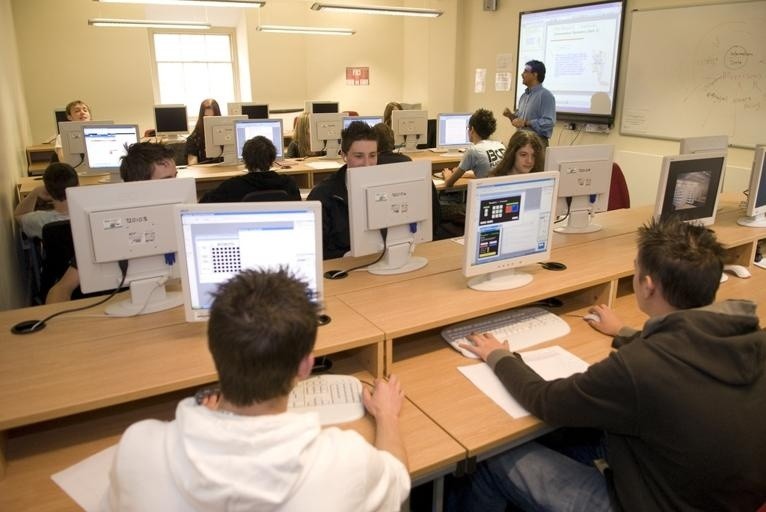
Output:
<box><xmin>736</xmin><ymin>144</ymin><xmax>766</xmax><ymax>229</ymax></box>
<box><xmin>653</xmin><ymin>151</ymin><xmax>728</xmax><ymax>226</ymax></box>
<box><xmin>227</xmin><ymin>102</ymin><xmax>256</xmax><ymax>116</ymax></box>
<box><xmin>341</xmin><ymin>115</ymin><xmax>385</xmax><ymax>135</ymax></box>
<box><xmin>308</xmin><ymin>112</ymin><xmax>350</xmax><ymax>160</ymax></box>
<box><xmin>678</xmin><ymin>135</ymin><xmax>731</xmax><ymax>194</ymax></box>
<box><xmin>461</xmin><ymin>170</ymin><xmax>560</xmax><ymax>290</ymax></box>
<box><xmin>173</xmin><ymin>200</ymin><xmax>325</xmax><ymax>323</ymax></box>
<box><xmin>345</xmin><ymin>159</ymin><xmax>433</xmax><ymax>275</ymax></box>
<box><xmin>436</xmin><ymin>112</ymin><xmax>474</xmax><ymax>151</ymax></box>
<box><xmin>55</xmin><ymin>109</ymin><xmax>72</xmax><ymax>135</ymax></box>
<box><xmin>64</xmin><ymin>176</ymin><xmax>198</xmax><ymax>318</ymax></box>
<box><xmin>57</xmin><ymin>120</ymin><xmax>115</xmax><ymax>176</ymax></box>
<box><xmin>80</xmin><ymin>124</ymin><xmax>140</xmax><ymax>172</ymax></box>
<box><xmin>543</xmin><ymin>143</ymin><xmax>617</xmax><ymax>234</ymax></box>
<box><xmin>241</xmin><ymin>104</ymin><xmax>269</xmax><ymax>119</ymax></box>
<box><xmin>416</xmin><ymin>117</ymin><xmax>436</xmax><ymax>149</ymax></box>
<box><xmin>391</xmin><ymin>109</ymin><xmax>428</xmax><ymax>151</ymax></box>
<box><xmin>233</xmin><ymin>119</ymin><xmax>284</xmax><ymax>159</ymax></box>
<box><xmin>305</xmin><ymin>101</ymin><xmax>339</xmax><ymax>115</ymax></box>
<box><xmin>154</xmin><ymin>105</ymin><xmax>189</xmax><ymax>143</ymax></box>
<box><xmin>203</xmin><ymin>115</ymin><xmax>249</xmax><ymax>167</ymax></box>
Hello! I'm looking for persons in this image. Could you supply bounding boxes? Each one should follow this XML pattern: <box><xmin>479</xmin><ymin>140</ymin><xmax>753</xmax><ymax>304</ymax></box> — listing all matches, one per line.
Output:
<box><xmin>383</xmin><ymin>103</ymin><xmax>403</xmax><ymax>127</ymax></box>
<box><xmin>185</xmin><ymin>99</ymin><xmax>224</xmax><ymax>165</ymax></box>
<box><xmin>112</xmin><ymin>264</ymin><xmax>412</xmax><ymax>512</ymax></box>
<box><xmin>45</xmin><ymin>139</ymin><xmax>178</xmax><ymax>302</ymax></box>
<box><xmin>460</xmin><ymin>215</ymin><xmax>766</xmax><ymax>511</ymax></box>
<box><xmin>442</xmin><ymin>108</ymin><xmax>507</xmax><ymax>235</ymax></box>
<box><xmin>503</xmin><ymin>59</ymin><xmax>556</xmax><ymax>147</ymax></box>
<box><xmin>305</xmin><ymin>120</ymin><xmax>440</xmax><ymax>261</ymax></box>
<box><xmin>287</xmin><ymin>112</ymin><xmax>327</xmax><ymax>158</ymax></box>
<box><xmin>54</xmin><ymin>100</ymin><xmax>91</xmax><ymax>163</ymax></box>
<box><xmin>486</xmin><ymin>131</ymin><xmax>545</xmax><ymax>179</ymax></box>
<box><xmin>199</xmin><ymin>136</ymin><xmax>302</xmax><ymax>203</ymax></box>
<box><xmin>14</xmin><ymin>163</ymin><xmax>79</xmax><ymax>238</ymax></box>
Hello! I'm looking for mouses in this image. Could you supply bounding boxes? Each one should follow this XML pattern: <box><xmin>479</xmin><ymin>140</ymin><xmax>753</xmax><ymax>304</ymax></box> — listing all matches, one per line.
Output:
<box><xmin>584</xmin><ymin>313</ymin><xmax>600</xmax><ymax>323</ymax></box>
<box><xmin>719</xmin><ymin>272</ymin><xmax>729</xmax><ymax>283</ymax></box>
<box><xmin>721</xmin><ymin>263</ymin><xmax>753</xmax><ymax>279</ymax></box>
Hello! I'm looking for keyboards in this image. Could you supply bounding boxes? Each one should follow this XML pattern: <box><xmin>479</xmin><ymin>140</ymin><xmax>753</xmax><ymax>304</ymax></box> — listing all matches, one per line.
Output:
<box><xmin>175</xmin><ymin>373</ymin><xmax>367</xmax><ymax>426</ymax></box>
<box><xmin>440</xmin><ymin>306</ymin><xmax>571</xmax><ymax>359</ymax></box>
<box><xmin>433</xmin><ymin>171</ymin><xmax>444</xmax><ymax>180</ymax></box>
